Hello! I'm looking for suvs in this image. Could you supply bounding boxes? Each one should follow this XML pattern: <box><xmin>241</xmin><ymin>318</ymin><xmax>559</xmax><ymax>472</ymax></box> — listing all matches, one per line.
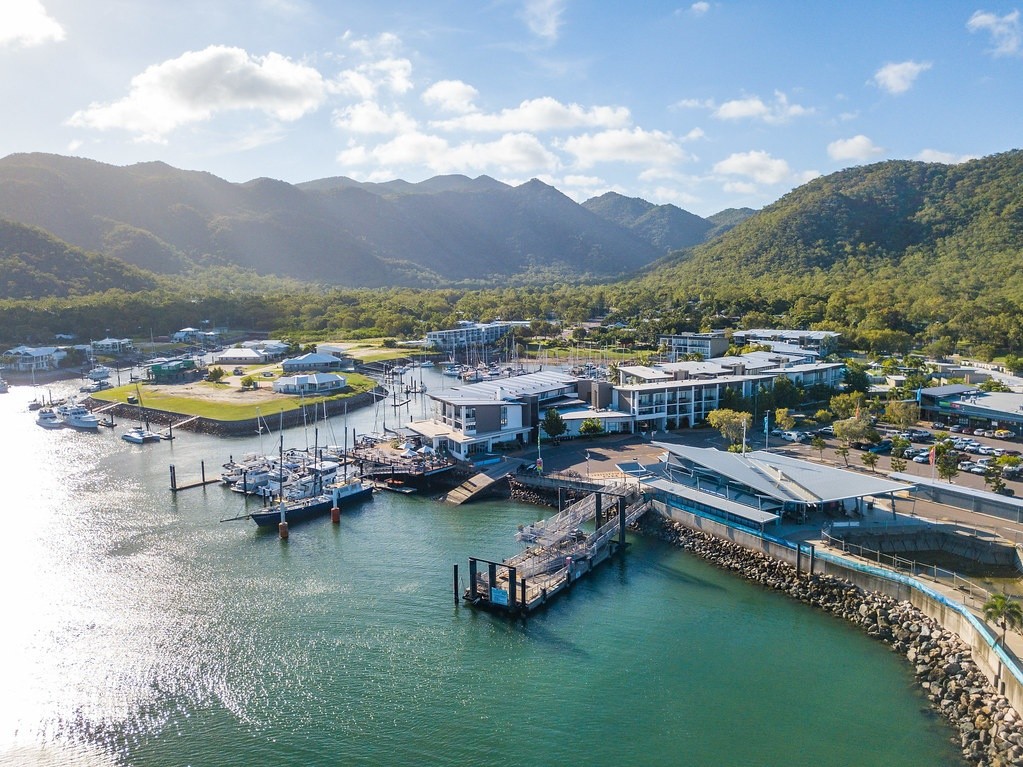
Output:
<box><xmin>995</xmin><ymin>430</ymin><xmax>1017</xmax><ymax>439</ymax></box>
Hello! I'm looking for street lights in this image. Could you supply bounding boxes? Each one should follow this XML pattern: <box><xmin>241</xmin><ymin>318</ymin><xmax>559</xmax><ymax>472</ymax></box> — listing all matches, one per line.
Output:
<box><xmin>765</xmin><ymin>410</ymin><xmax>771</xmax><ymax>451</ymax></box>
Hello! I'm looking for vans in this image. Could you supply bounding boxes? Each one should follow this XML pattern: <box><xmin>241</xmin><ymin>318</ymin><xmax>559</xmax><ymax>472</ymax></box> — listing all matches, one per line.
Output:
<box><xmin>931</xmin><ymin>422</ymin><xmax>944</xmax><ymax>429</ymax></box>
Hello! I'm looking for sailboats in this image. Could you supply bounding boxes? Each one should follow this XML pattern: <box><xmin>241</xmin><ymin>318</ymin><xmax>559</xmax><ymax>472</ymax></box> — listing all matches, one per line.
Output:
<box><xmin>411</xmin><ymin>367</ymin><xmax>427</xmax><ymax>393</ymax></box>
<box><xmin>439</xmin><ymin>334</ymin><xmax>611</xmax><ymax>382</ymax></box>
<box><xmin>222</xmin><ymin>389</ymin><xmax>347</xmax><ymax>501</ymax></box>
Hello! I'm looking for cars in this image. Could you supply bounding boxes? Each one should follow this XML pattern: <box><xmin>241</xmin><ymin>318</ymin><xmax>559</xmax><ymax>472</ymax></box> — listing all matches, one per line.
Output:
<box><xmin>860</xmin><ymin>430</ymin><xmax>1023</xmax><ymax>476</ymax></box>
<box><xmin>962</xmin><ymin>427</ymin><xmax>975</xmax><ymax>434</ymax></box>
<box><xmin>818</xmin><ymin>427</ymin><xmax>835</xmax><ymax>436</ymax></box>
<box><xmin>771</xmin><ymin>428</ymin><xmax>782</xmax><ymax>436</ymax></box>
<box><xmin>973</xmin><ymin>428</ymin><xmax>986</xmax><ymax>436</ymax></box>
<box><xmin>984</xmin><ymin>430</ymin><xmax>995</xmax><ymax>438</ymax></box>
<box><xmin>949</xmin><ymin>424</ymin><xmax>963</xmax><ymax>432</ymax></box>
<box><xmin>798</xmin><ymin>431</ymin><xmax>815</xmax><ymax>439</ymax></box>
<box><xmin>781</xmin><ymin>431</ymin><xmax>795</xmax><ymax>442</ymax></box>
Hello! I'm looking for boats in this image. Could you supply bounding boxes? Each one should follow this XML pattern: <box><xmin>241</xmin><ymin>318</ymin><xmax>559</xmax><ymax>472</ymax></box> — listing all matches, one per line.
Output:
<box><xmin>60</xmin><ymin>403</ymin><xmax>101</xmax><ymax>428</ymax></box>
<box><xmin>78</xmin><ymin>381</ymin><xmax>113</xmax><ymax>393</ymax></box>
<box><xmin>390</xmin><ymin>364</ymin><xmax>406</xmax><ymax>375</ymax></box>
<box><xmin>36</xmin><ymin>407</ymin><xmax>64</xmax><ymax>428</ymax></box>
<box><xmin>121</xmin><ymin>377</ymin><xmax>161</xmax><ymax>444</ymax></box>
<box><xmin>420</xmin><ymin>360</ymin><xmax>436</xmax><ymax>367</ymax></box>
<box><xmin>88</xmin><ymin>366</ymin><xmax>111</xmax><ymax>381</ymax></box>
